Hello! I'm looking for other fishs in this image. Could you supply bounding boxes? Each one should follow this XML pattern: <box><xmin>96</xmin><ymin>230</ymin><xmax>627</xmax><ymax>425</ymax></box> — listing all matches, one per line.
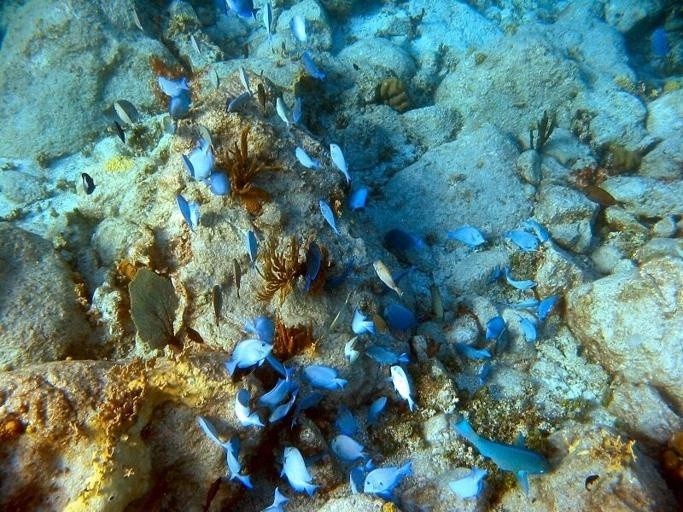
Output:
<box><xmin>196</xmin><ymin>307</ymin><xmax>412</xmax><ymax>509</ymax></box>
<box><xmin>365</xmin><ymin>257</ymin><xmax>419</xmax><ymax>416</ymax></box>
<box><xmin>158</xmin><ymin>0</ymin><xmax>375</xmax><ymax>316</ymax></box>
